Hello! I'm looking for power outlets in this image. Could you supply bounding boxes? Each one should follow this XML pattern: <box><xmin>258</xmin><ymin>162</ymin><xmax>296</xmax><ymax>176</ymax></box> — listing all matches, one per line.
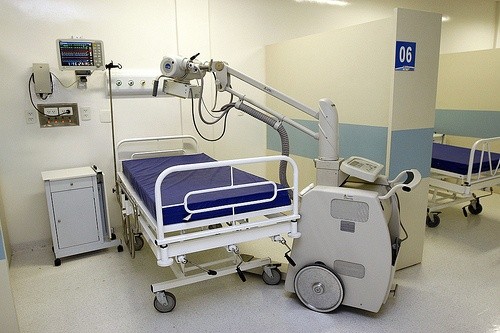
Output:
<box><xmin>59</xmin><ymin>107</ymin><xmax>73</xmax><ymax>116</ymax></box>
<box><xmin>43</xmin><ymin>108</ymin><xmax>58</xmax><ymax>117</ymax></box>
<box><xmin>24</xmin><ymin>110</ymin><xmax>37</xmax><ymax>124</ymax></box>
<box><xmin>80</xmin><ymin>107</ymin><xmax>91</xmax><ymax>122</ymax></box>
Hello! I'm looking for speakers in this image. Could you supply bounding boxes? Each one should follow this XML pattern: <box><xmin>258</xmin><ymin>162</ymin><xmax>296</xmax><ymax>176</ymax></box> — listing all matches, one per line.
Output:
<box><xmin>32</xmin><ymin>63</ymin><xmax>52</xmax><ymax>93</ymax></box>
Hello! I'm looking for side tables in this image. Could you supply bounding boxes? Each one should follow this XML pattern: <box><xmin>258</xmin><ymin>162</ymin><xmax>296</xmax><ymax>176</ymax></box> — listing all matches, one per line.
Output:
<box><xmin>41</xmin><ymin>164</ymin><xmax>123</xmax><ymax>266</ymax></box>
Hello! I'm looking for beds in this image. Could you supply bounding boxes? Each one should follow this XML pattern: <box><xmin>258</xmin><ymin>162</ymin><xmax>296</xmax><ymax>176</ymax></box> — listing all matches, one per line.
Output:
<box><xmin>427</xmin><ymin>134</ymin><xmax>500</xmax><ymax>228</ymax></box>
<box><xmin>112</xmin><ymin>136</ymin><xmax>301</xmax><ymax>314</ymax></box>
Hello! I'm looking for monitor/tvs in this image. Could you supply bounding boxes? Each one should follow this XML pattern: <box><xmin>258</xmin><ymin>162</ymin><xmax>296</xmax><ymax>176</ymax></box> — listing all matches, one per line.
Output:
<box><xmin>56</xmin><ymin>38</ymin><xmax>105</xmax><ymax>71</ymax></box>
